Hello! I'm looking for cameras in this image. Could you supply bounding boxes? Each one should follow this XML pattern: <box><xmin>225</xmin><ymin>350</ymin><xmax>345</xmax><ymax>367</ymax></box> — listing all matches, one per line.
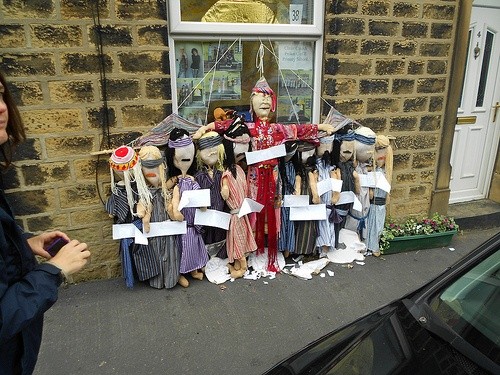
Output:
<box><xmin>46</xmin><ymin>238</ymin><xmax>68</xmax><ymax>257</ymax></box>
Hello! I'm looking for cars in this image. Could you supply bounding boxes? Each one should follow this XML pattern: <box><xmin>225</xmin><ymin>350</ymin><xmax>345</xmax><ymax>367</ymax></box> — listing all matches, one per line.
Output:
<box><xmin>257</xmin><ymin>232</ymin><xmax>500</xmax><ymax>375</ymax></box>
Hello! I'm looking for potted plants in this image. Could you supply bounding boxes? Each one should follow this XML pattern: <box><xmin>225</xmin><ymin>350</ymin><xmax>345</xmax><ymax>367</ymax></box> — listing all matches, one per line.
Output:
<box><xmin>378</xmin><ymin>212</ymin><xmax>459</xmax><ymax>254</ymax></box>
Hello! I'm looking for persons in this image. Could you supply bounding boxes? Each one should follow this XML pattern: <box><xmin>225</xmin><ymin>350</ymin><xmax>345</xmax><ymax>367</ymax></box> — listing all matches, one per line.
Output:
<box><xmin>102</xmin><ymin>76</ymin><xmax>397</xmax><ymax>289</ymax></box>
<box><xmin>177</xmin><ymin>47</ymin><xmax>188</xmax><ymax>78</ymax></box>
<box><xmin>0</xmin><ymin>71</ymin><xmax>90</xmax><ymax>375</ymax></box>
<box><xmin>190</xmin><ymin>48</ymin><xmax>200</xmax><ymax>78</ymax></box>
